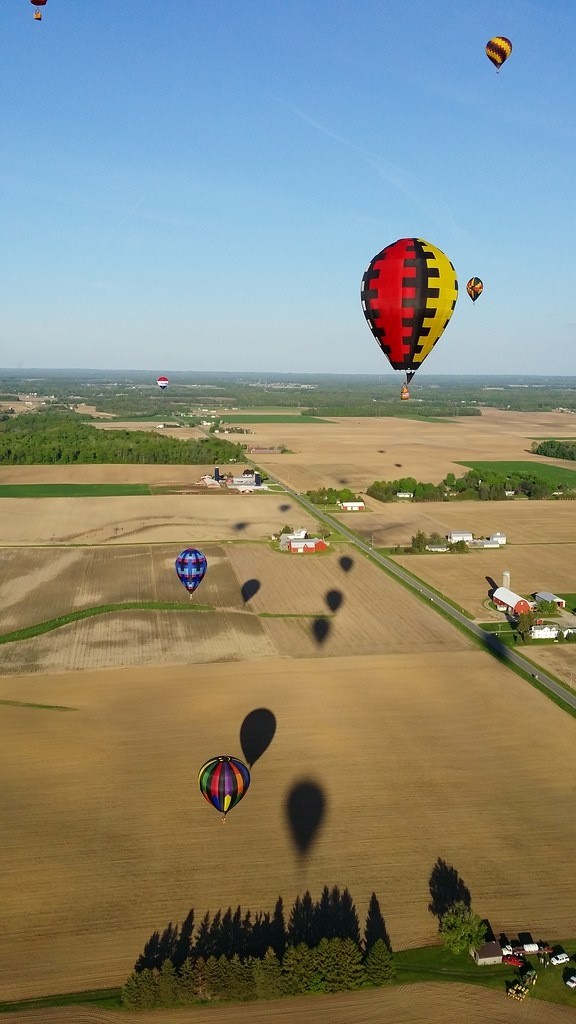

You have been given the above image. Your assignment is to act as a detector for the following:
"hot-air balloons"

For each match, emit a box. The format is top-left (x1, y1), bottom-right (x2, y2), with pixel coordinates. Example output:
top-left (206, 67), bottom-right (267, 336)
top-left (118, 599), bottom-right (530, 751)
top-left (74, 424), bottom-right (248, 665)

top-left (485, 36), bottom-right (512, 74)
top-left (158, 377), bottom-right (169, 394)
top-left (360, 238), bottom-right (459, 400)
top-left (466, 277), bottom-right (484, 305)
top-left (175, 548), bottom-right (208, 602)
top-left (30, 0), bottom-right (48, 21)
top-left (197, 755), bottom-right (251, 823)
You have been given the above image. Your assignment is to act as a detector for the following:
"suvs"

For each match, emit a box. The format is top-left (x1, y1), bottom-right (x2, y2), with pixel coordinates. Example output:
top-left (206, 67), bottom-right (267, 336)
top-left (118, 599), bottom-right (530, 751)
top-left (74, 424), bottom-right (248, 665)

top-left (550, 953), bottom-right (570, 966)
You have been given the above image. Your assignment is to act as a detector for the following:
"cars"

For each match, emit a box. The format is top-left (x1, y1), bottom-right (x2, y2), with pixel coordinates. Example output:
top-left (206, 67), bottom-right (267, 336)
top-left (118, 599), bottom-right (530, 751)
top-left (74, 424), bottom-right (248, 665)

top-left (503, 955), bottom-right (524, 968)
top-left (566, 975), bottom-right (576, 989)
